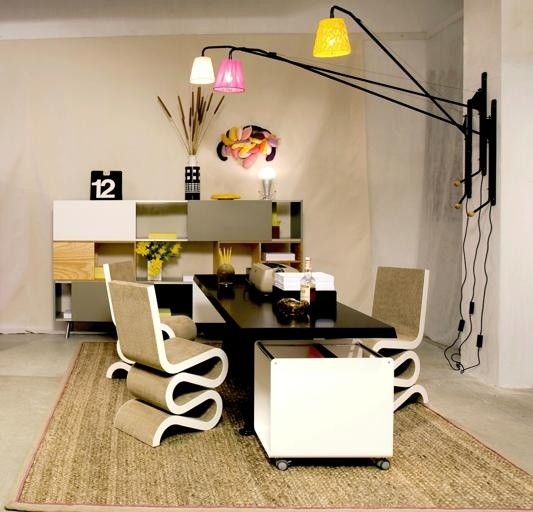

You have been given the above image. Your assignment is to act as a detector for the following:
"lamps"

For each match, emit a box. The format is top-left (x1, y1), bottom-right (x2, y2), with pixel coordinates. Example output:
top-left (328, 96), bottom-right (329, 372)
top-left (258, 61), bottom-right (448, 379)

top-left (190, 44), bottom-right (497, 217)
top-left (312, 4), bottom-right (471, 201)
top-left (212, 46), bottom-right (489, 187)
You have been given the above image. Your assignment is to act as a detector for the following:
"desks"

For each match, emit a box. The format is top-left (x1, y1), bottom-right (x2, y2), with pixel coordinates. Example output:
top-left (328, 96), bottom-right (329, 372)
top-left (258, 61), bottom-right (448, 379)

top-left (191, 275), bottom-right (394, 436)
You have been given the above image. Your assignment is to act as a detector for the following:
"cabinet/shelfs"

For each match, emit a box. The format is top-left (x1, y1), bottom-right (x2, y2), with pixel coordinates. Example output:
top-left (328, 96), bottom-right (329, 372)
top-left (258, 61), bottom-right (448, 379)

top-left (253, 340), bottom-right (395, 472)
top-left (50, 199), bottom-right (302, 340)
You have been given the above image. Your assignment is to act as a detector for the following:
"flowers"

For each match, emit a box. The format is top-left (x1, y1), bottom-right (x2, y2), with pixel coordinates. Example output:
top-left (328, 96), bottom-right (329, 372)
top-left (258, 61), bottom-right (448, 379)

top-left (134, 240), bottom-right (183, 277)
top-left (217, 122), bottom-right (279, 169)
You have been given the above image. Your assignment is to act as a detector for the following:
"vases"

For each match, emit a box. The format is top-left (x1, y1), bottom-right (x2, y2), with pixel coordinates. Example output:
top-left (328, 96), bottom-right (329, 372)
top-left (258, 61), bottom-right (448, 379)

top-left (147, 260), bottom-right (163, 283)
top-left (239, 153), bottom-right (257, 199)
top-left (183, 154), bottom-right (201, 199)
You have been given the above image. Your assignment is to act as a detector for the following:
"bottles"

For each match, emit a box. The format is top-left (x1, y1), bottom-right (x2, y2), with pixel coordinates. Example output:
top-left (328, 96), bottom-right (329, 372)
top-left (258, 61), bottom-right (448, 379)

top-left (299, 256), bottom-right (316, 309)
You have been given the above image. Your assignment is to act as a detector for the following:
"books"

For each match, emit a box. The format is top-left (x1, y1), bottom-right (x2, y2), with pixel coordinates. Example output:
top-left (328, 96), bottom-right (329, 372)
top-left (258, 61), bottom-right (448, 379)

top-left (62, 309), bottom-right (70, 318)
top-left (261, 251), bottom-right (296, 262)
top-left (148, 231), bottom-right (177, 239)
top-left (158, 307), bottom-right (171, 316)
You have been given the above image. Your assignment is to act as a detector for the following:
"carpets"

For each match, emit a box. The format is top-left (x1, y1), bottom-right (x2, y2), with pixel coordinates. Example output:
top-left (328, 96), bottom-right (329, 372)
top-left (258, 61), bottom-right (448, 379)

top-left (4, 341), bottom-right (533, 511)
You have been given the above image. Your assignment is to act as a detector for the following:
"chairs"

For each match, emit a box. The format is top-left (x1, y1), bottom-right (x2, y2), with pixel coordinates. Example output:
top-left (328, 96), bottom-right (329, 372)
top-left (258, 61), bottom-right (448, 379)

top-left (102, 260), bottom-right (198, 378)
top-left (333, 265), bottom-right (430, 413)
top-left (105, 279), bottom-right (229, 448)
